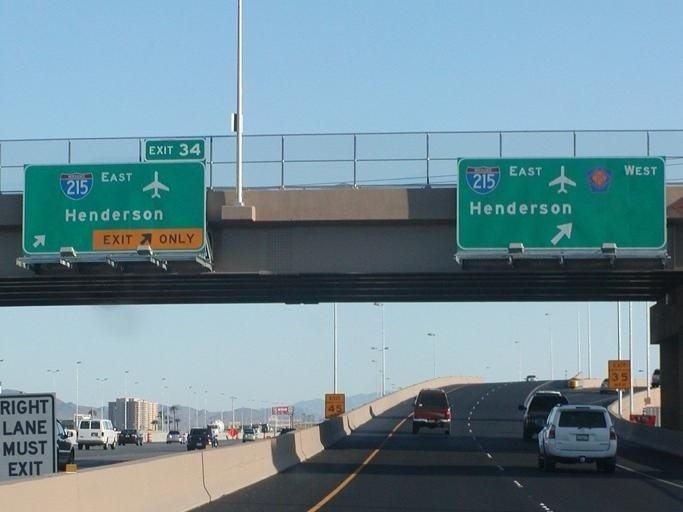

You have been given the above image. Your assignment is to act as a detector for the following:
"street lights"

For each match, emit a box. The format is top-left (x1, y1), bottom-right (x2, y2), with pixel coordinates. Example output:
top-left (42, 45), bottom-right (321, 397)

top-left (160, 378), bottom-right (293, 437)
top-left (125, 370), bottom-right (130, 430)
top-left (96, 377), bottom-right (109, 419)
top-left (46, 369), bottom-right (61, 393)
top-left (76, 359), bottom-right (82, 423)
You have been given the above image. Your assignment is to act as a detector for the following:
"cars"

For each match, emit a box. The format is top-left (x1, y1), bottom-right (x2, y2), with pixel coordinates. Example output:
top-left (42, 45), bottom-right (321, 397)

top-left (242, 428), bottom-right (256, 443)
top-left (651, 369), bottom-right (661, 388)
top-left (55, 417), bottom-right (76, 470)
top-left (599, 378), bottom-right (625, 395)
top-left (166, 430), bottom-right (186, 445)
top-left (118, 428), bottom-right (144, 446)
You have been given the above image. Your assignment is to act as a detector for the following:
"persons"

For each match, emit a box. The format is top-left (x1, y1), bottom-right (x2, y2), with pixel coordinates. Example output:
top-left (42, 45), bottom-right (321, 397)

top-left (136, 425), bottom-right (144, 448)
top-left (210, 430), bottom-right (217, 448)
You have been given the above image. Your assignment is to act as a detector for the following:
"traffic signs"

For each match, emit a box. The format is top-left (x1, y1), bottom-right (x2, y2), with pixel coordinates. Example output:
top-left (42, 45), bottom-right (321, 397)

top-left (454, 154), bottom-right (668, 258)
top-left (21, 160), bottom-right (208, 261)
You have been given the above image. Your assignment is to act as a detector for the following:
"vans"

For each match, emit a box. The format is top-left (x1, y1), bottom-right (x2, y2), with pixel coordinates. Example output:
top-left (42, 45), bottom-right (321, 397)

top-left (526, 375), bottom-right (537, 382)
top-left (186, 427), bottom-right (219, 451)
top-left (411, 388), bottom-right (451, 435)
top-left (77, 418), bottom-right (118, 451)
top-left (517, 389), bottom-right (568, 440)
top-left (536, 403), bottom-right (617, 473)
